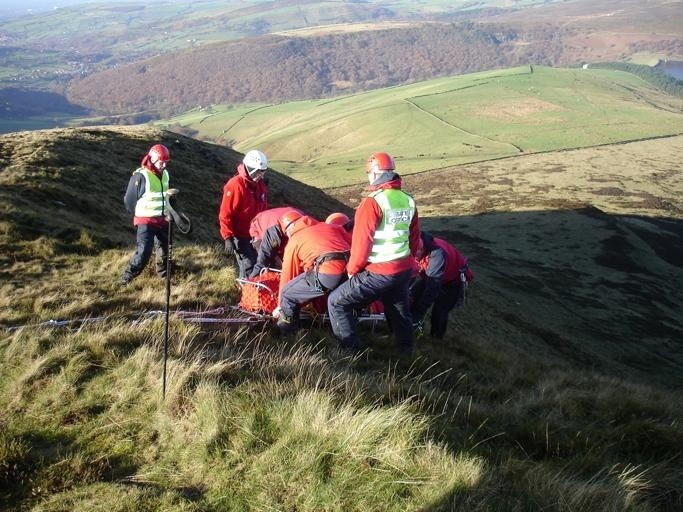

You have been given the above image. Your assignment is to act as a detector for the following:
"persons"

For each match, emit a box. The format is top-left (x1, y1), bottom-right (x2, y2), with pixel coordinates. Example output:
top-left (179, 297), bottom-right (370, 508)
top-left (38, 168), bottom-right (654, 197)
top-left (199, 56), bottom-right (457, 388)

top-left (120, 144), bottom-right (184, 283)
top-left (328, 152), bottom-right (419, 355)
top-left (218, 150), bottom-right (268, 278)
top-left (416, 231), bottom-right (473, 340)
top-left (247, 207), bottom-right (424, 343)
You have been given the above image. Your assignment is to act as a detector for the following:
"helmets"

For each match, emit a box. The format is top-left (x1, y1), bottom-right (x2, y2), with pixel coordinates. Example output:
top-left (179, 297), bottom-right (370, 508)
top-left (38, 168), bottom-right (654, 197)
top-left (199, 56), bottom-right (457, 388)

top-left (280, 212), bottom-right (303, 235)
top-left (324, 213), bottom-right (352, 227)
top-left (147, 144), bottom-right (170, 164)
top-left (366, 152), bottom-right (395, 173)
top-left (242, 149), bottom-right (268, 171)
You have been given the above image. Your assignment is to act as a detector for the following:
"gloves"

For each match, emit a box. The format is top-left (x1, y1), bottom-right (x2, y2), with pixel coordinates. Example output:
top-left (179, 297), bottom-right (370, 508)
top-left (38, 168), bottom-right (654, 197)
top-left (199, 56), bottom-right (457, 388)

top-left (224, 239), bottom-right (237, 256)
